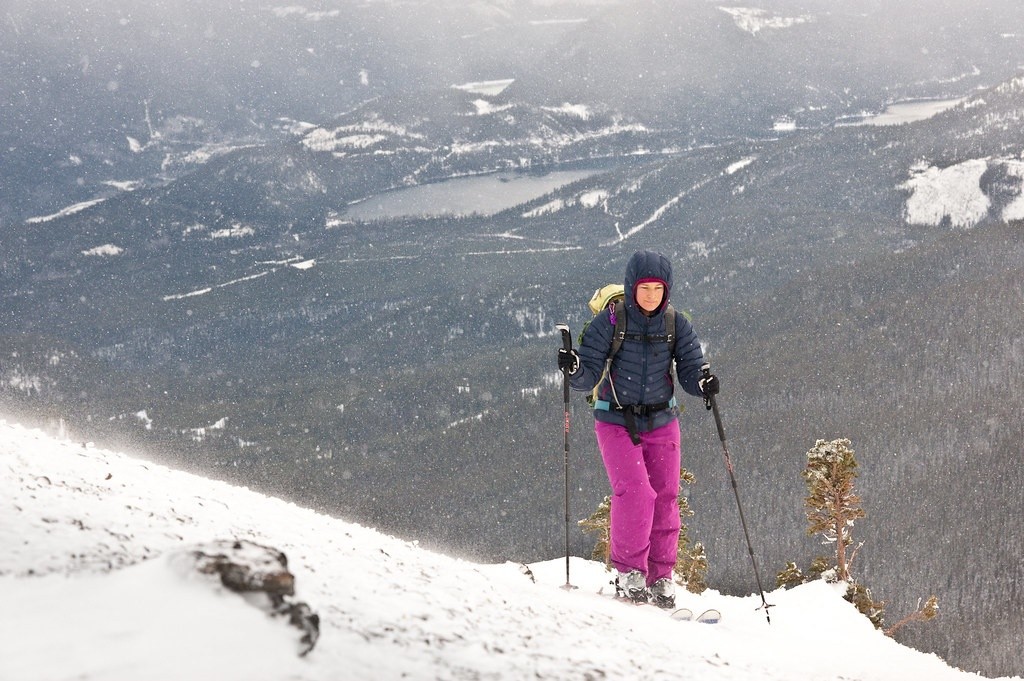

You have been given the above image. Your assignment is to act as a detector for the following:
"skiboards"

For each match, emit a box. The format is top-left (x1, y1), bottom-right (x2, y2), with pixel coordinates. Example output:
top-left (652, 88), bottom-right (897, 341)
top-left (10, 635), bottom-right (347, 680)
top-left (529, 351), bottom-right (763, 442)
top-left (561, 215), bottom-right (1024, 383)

top-left (600, 569), bottom-right (724, 624)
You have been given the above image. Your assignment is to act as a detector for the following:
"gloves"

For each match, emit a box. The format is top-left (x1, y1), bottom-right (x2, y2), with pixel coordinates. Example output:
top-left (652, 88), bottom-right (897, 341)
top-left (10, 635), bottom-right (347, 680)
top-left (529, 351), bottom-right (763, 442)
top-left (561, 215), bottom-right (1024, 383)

top-left (557, 348), bottom-right (582, 377)
top-left (698, 372), bottom-right (719, 396)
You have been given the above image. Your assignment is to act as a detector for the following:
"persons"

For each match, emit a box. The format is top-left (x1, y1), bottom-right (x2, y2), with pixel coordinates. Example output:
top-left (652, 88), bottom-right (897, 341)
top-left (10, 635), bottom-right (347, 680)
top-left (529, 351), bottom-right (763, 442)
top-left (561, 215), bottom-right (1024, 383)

top-left (557, 249), bottom-right (719, 606)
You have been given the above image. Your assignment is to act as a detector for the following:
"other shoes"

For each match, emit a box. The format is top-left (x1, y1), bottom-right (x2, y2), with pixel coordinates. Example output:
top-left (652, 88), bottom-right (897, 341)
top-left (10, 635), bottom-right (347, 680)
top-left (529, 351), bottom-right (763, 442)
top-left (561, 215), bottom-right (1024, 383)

top-left (616, 575), bottom-right (648, 603)
top-left (648, 580), bottom-right (675, 609)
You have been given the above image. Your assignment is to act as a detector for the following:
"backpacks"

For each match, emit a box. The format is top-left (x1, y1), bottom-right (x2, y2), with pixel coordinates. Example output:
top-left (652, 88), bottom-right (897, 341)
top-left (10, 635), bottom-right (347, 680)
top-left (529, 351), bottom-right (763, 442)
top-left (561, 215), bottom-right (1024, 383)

top-left (588, 285), bottom-right (676, 381)
